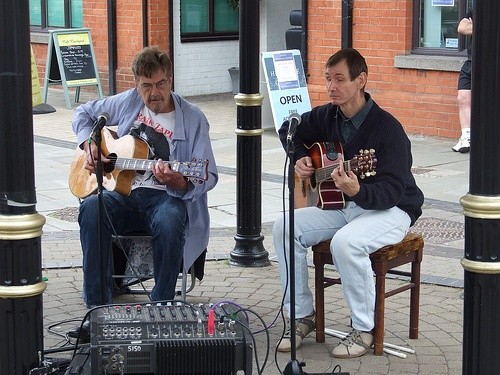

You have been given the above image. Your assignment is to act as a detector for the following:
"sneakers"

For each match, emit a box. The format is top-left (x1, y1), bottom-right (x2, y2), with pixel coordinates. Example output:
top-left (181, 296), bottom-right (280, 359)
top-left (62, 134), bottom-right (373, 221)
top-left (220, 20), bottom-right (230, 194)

top-left (275, 310), bottom-right (315, 351)
top-left (329, 329), bottom-right (374, 357)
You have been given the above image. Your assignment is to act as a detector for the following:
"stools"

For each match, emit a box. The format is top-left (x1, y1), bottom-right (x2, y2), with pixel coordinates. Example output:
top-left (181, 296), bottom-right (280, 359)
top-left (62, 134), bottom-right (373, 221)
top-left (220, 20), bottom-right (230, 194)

top-left (311, 232), bottom-right (424, 357)
top-left (111, 230), bottom-right (195, 306)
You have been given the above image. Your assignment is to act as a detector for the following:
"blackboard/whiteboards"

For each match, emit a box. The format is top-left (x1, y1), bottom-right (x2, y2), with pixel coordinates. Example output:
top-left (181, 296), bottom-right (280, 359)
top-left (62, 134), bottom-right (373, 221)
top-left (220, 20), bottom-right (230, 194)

top-left (45, 27), bottom-right (101, 87)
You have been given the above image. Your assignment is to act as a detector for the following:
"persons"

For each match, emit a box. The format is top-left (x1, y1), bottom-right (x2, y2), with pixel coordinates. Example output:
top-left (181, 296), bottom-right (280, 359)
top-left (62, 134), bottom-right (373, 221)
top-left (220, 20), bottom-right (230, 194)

top-left (275, 48), bottom-right (425, 358)
top-left (452, 11), bottom-right (472, 153)
top-left (67, 45), bottom-right (219, 343)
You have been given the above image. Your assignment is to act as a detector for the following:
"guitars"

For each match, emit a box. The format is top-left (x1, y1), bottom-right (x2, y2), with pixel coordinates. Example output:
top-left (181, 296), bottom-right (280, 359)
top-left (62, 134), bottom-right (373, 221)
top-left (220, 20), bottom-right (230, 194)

top-left (293, 139), bottom-right (378, 210)
top-left (69, 127), bottom-right (209, 199)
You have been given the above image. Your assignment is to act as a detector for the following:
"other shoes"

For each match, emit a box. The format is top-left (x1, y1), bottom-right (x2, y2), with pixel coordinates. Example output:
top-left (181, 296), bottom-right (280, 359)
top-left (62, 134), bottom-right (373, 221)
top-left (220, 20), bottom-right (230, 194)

top-left (452, 143), bottom-right (462, 152)
top-left (67, 319), bottom-right (91, 338)
top-left (458, 136), bottom-right (470, 152)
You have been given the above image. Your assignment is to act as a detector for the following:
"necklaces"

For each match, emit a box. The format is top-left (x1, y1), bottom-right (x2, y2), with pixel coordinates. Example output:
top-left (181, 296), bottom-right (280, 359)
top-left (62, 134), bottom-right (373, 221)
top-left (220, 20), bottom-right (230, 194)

top-left (150, 111), bottom-right (158, 125)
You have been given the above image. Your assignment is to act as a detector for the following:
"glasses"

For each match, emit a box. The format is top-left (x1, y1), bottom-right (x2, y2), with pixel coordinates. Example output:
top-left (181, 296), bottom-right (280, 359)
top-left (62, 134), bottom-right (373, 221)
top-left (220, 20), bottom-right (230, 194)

top-left (137, 78), bottom-right (169, 93)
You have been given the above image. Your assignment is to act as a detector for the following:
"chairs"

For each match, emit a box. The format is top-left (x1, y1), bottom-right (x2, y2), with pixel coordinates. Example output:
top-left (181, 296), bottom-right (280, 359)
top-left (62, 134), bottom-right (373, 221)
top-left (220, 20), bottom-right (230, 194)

top-left (286, 10), bottom-right (302, 50)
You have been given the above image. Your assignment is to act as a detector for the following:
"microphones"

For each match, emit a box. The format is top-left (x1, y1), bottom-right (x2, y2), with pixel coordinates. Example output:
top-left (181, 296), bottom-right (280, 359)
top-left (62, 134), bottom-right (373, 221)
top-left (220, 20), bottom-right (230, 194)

top-left (287, 113), bottom-right (302, 145)
top-left (90, 112), bottom-right (110, 139)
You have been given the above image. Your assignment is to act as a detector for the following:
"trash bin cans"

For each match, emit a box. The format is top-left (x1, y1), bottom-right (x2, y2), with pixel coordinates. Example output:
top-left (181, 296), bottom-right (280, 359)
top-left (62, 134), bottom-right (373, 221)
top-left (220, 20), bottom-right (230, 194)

top-left (228, 66), bottom-right (240, 95)
